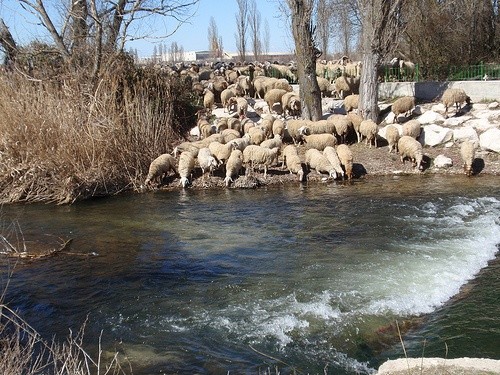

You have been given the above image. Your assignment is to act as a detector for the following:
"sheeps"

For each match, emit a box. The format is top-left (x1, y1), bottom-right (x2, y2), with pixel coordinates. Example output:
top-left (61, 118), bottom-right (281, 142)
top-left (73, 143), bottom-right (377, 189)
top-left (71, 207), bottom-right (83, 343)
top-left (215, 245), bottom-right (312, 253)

top-left (142, 54), bottom-right (424, 188)
top-left (459, 140), bottom-right (476, 178)
top-left (437, 88), bottom-right (471, 116)
